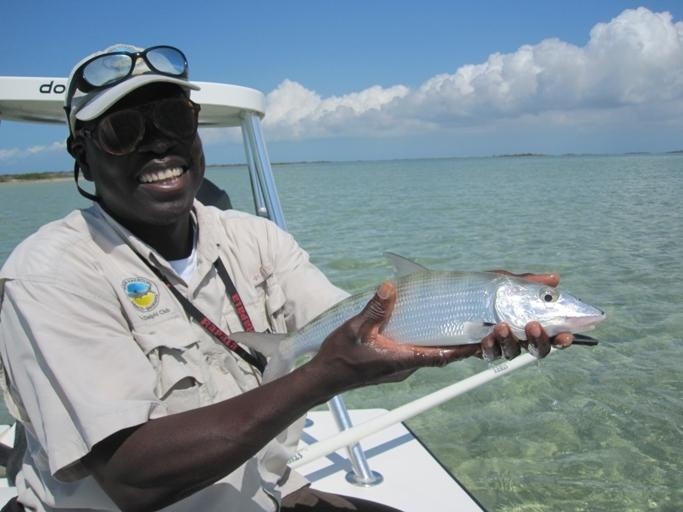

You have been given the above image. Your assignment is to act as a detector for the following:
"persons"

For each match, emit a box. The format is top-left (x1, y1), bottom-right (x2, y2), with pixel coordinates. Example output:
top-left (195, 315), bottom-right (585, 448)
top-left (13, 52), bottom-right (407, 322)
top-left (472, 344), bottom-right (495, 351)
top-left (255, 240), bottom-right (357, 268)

top-left (0, 44), bottom-right (572, 511)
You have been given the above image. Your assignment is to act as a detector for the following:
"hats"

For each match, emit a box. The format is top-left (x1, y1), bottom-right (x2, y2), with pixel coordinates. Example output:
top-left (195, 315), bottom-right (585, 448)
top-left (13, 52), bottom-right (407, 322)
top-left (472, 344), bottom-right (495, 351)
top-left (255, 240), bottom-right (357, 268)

top-left (64, 43), bottom-right (200, 138)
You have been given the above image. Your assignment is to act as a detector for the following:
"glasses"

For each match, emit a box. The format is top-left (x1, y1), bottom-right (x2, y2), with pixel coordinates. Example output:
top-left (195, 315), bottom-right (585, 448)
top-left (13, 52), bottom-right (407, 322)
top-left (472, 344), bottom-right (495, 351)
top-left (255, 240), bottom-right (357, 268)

top-left (66, 44), bottom-right (187, 111)
top-left (74, 99), bottom-right (200, 155)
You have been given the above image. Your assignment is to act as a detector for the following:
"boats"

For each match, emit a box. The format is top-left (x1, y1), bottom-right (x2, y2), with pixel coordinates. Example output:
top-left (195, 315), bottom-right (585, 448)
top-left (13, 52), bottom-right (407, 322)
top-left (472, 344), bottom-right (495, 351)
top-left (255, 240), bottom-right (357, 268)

top-left (0, 74), bottom-right (486, 512)
